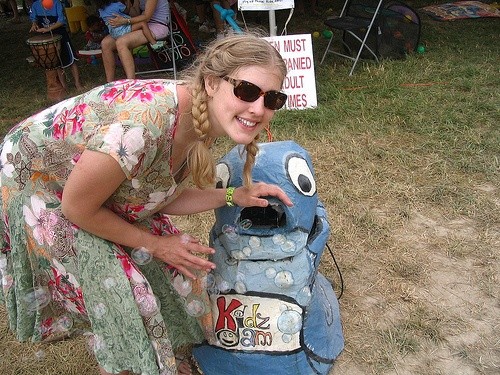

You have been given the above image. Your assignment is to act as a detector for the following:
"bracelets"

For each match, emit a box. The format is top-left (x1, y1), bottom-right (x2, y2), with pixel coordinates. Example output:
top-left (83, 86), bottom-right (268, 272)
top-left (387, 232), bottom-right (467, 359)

top-left (127, 19), bottom-right (130, 25)
top-left (225, 186), bottom-right (236, 208)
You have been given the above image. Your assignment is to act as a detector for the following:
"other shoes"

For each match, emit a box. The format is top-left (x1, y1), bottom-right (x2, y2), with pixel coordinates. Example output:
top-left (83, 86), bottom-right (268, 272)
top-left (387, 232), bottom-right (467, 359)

top-left (150, 40), bottom-right (167, 50)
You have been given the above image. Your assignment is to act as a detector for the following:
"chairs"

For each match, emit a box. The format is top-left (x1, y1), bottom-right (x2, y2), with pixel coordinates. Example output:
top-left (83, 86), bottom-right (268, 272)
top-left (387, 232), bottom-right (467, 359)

top-left (135, 9), bottom-right (181, 80)
top-left (319, 0), bottom-right (383, 77)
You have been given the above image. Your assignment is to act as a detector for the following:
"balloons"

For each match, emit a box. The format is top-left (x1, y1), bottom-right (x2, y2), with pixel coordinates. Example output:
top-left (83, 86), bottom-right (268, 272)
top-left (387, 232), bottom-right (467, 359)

top-left (214, 4), bottom-right (242, 35)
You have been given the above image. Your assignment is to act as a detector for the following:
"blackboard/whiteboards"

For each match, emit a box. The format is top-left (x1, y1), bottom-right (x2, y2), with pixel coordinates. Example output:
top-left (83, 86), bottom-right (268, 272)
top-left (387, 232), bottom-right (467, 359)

top-left (150, 6), bottom-right (195, 73)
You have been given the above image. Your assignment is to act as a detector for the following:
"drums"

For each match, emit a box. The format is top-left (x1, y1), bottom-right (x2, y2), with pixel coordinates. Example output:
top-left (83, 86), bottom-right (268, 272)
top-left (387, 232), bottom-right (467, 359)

top-left (26, 34), bottom-right (70, 101)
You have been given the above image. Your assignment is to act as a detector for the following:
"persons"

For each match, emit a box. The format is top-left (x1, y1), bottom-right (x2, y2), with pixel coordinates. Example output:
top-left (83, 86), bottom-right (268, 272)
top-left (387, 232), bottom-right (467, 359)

top-left (84, 15), bottom-right (101, 53)
top-left (196, 0), bottom-right (225, 40)
top-left (100, 0), bottom-right (172, 85)
top-left (96, 0), bottom-right (168, 53)
top-left (30, 0), bottom-right (86, 93)
top-left (0, 32), bottom-right (292, 375)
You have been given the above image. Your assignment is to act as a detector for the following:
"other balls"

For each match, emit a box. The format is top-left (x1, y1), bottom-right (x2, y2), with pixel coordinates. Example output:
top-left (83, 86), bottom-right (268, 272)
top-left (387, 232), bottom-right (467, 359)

top-left (325, 31), bottom-right (333, 39)
top-left (313, 32), bottom-right (320, 38)
top-left (323, 29), bottom-right (328, 35)
top-left (417, 45), bottom-right (425, 54)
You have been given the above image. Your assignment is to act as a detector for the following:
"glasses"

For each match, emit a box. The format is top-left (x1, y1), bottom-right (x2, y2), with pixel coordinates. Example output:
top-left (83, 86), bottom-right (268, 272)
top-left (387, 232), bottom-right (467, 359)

top-left (218, 74), bottom-right (288, 110)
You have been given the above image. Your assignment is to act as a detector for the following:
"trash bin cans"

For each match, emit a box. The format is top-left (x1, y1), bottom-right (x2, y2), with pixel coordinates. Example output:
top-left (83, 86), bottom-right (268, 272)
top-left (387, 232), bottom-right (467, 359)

top-left (190, 140), bottom-right (345, 375)
